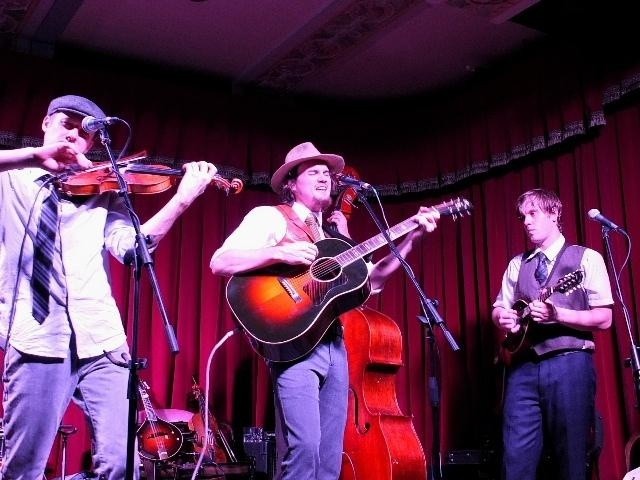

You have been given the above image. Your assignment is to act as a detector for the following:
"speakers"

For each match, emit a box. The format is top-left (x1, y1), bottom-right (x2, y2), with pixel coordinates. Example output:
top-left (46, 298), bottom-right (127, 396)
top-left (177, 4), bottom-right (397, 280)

top-left (243, 433), bottom-right (276, 480)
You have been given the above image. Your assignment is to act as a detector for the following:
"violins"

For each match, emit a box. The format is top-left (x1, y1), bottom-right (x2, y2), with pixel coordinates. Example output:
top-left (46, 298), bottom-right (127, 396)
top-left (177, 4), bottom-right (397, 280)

top-left (58, 163), bottom-right (242, 197)
top-left (188, 376), bottom-right (230, 468)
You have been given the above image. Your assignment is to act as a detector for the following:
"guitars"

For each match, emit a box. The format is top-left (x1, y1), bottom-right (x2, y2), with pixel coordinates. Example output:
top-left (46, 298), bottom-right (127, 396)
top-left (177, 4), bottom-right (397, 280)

top-left (499, 272), bottom-right (584, 354)
top-left (226, 196), bottom-right (475, 361)
top-left (137, 379), bottom-right (183, 461)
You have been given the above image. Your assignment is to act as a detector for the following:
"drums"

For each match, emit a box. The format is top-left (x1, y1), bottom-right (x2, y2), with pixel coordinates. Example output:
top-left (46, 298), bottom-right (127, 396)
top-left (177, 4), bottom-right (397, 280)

top-left (135, 409), bottom-right (198, 479)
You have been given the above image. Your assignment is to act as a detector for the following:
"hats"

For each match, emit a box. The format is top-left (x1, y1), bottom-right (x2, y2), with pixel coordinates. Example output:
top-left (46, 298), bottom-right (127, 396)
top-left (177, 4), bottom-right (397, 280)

top-left (47, 95), bottom-right (107, 120)
top-left (271, 142), bottom-right (345, 195)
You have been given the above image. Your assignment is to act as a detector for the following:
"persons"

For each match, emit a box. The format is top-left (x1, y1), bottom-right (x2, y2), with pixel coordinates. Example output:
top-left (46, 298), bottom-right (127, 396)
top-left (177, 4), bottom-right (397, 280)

top-left (325, 208), bottom-right (354, 243)
top-left (488, 188), bottom-right (617, 480)
top-left (210, 140), bottom-right (441, 480)
top-left (0, 94), bottom-right (220, 480)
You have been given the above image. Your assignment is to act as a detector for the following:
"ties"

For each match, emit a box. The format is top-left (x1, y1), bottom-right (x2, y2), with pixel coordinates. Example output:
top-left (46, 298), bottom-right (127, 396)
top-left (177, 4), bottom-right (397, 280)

top-left (534, 252), bottom-right (548, 285)
top-left (305, 213), bottom-right (321, 241)
top-left (32, 174), bottom-right (68, 325)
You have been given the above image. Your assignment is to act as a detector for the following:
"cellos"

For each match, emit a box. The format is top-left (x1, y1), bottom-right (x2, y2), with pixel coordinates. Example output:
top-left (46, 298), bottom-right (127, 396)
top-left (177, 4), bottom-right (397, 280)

top-left (323, 167), bottom-right (426, 480)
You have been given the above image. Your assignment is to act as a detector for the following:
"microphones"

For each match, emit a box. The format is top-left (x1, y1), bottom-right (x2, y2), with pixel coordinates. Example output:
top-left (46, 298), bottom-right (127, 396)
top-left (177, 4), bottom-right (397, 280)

top-left (81, 116), bottom-right (119, 134)
top-left (331, 172), bottom-right (371, 189)
top-left (587, 209), bottom-right (627, 235)
